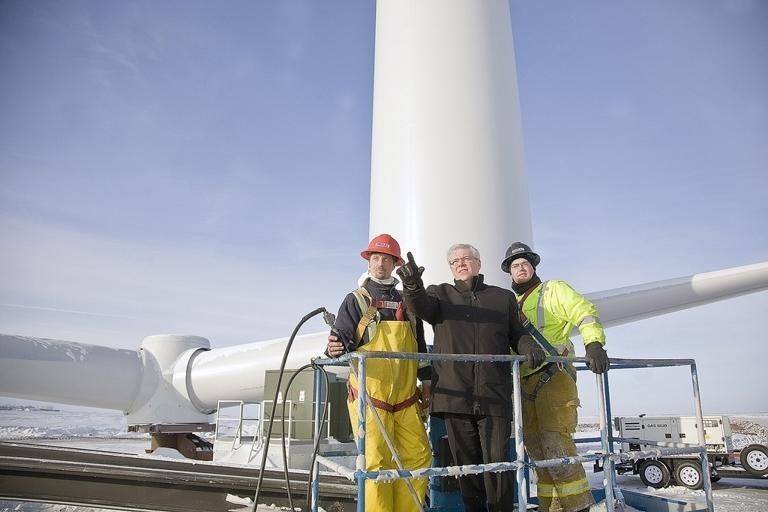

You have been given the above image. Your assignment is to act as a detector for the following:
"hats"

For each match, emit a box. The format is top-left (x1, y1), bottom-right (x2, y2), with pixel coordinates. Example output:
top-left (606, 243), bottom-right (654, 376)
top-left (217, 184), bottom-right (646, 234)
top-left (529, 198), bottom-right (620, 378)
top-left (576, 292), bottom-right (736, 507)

top-left (360, 234), bottom-right (405, 267)
top-left (500, 242), bottom-right (541, 274)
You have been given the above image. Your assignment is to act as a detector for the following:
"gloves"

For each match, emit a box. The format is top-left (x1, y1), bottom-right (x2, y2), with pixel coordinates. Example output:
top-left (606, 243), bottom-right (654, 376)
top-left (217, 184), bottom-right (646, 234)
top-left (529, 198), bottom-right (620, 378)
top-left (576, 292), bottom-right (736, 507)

top-left (396, 252), bottom-right (425, 291)
top-left (584, 341), bottom-right (610, 375)
top-left (517, 334), bottom-right (547, 370)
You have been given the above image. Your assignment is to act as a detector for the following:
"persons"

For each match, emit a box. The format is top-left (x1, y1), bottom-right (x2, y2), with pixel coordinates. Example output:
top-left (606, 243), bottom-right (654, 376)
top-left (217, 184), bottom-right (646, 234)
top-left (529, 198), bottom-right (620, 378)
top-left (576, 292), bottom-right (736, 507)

top-left (324, 233), bottom-right (435, 511)
top-left (500, 241), bottom-right (609, 511)
top-left (395, 242), bottom-right (547, 511)
